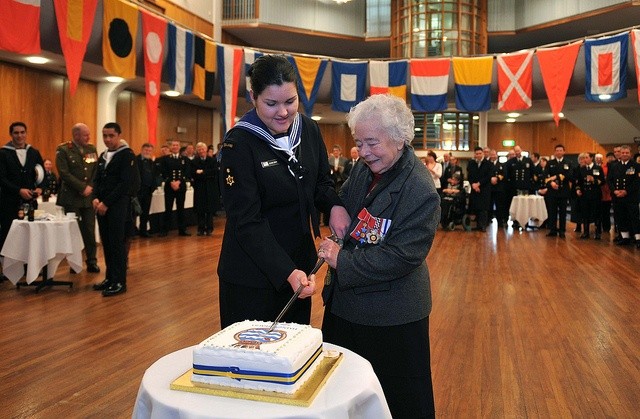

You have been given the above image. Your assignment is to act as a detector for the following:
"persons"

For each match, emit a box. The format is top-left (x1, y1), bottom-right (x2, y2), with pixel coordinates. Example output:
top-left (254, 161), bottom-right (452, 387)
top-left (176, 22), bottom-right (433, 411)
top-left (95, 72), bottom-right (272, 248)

top-left (0, 122), bottom-right (48, 257)
top-left (342, 146), bottom-right (359, 179)
top-left (136, 138), bottom-right (219, 238)
top-left (328, 144), bottom-right (349, 194)
top-left (55, 122), bottom-right (101, 273)
top-left (216, 54), bottom-right (352, 332)
top-left (317, 94), bottom-right (441, 418)
top-left (442, 145), bottom-right (545, 232)
top-left (90, 122), bottom-right (136, 297)
top-left (425, 150), bottom-right (443, 197)
top-left (545, 143), bottom-right (639, 246)
top-left (42, 159), bottom-right (57, 196)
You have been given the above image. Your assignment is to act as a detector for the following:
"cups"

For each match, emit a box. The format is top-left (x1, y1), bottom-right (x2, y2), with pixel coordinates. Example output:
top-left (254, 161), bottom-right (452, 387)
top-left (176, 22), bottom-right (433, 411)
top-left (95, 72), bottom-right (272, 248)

top-left (56, 206), bottom-right (63, 220)
top-left (67, 212), bottom-right (75, 221)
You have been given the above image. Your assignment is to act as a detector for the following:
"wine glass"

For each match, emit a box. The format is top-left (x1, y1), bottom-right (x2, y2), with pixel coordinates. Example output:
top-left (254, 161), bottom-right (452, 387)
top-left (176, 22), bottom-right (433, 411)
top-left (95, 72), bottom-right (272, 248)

top-left (23, 204), bottom-right (28, 219)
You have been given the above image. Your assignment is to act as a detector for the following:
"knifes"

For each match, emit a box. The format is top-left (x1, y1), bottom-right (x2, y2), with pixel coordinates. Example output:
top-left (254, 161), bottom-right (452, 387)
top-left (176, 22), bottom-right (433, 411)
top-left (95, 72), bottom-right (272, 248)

top-left (268, 233), bottom-right (344, 333)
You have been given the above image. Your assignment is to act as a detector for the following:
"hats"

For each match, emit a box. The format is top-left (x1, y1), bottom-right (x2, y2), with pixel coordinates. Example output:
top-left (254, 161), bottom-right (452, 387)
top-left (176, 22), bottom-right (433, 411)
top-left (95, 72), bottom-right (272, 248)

top-left (34, 163), bottom-right (45, 185)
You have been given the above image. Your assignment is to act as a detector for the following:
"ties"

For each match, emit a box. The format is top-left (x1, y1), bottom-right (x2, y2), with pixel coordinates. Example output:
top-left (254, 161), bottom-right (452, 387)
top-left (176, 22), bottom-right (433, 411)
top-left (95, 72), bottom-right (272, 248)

top-left (623, 162), bottom-right (625, 168)
top-left (559, 160), bottom-right (560, 165)
top-left (518, 158), bottom-right (521, 163)
top-left (174, 154), bottom-right (176, 161)
top-left (587, 166), bottom-right (590, 170)
top-left (478, 161), bottom-right (480, 168)
top-left (202, 158), bottom-right (205, 163)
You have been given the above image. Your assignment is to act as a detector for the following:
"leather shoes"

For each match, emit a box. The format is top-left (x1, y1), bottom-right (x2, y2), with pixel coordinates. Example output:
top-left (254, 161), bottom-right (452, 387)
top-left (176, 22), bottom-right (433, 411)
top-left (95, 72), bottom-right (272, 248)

top-left (617, 240), bottom-right (628, 245)
top-left (482, 225), bottom-right (486, 232)
top-left (546, 232), bottom-right (557, 236)
top-left (206, 231), bottom-right (212, 235)
top-left (559, 233), bottom-right (565, 238)
top-left (93, 279), bottom-right (109, 290)
top-left (580, 233), bottom-right (589, 238)
top-left (140, 232), bottom-right (152, 237)
top-left (102, 282), bottom-right (126, 296)
top-left (87, 265), bottom-right (100, 272)
top-left (635, 241), bottom-right (640, 248)
top-left (198, 231), bottom-right (204, 236)
top-left (179, 228), bottom-right (192, 236)
top-left (158, 230), bottom-right (169, 236)
top-left (594, 234), bottom-right (600, 239)
top-left (573, 228), bottom-right (581, 232)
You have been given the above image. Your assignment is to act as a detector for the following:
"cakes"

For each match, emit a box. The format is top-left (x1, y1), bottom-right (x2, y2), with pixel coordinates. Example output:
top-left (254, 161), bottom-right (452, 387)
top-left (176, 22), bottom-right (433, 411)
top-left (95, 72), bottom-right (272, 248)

top-left (190, 317), bottom-right (326, 395)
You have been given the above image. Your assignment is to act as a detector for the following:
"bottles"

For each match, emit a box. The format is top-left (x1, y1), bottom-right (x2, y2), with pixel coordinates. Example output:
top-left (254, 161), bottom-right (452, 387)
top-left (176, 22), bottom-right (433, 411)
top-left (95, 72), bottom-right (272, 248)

top-left (17, 199), bottom-right (24, 220)
top-left (27, 198), bottom-right (35, 221)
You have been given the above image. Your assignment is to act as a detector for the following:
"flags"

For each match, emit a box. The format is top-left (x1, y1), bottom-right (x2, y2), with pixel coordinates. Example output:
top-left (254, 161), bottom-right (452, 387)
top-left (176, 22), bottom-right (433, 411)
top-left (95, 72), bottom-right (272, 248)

top-left (168, 21), bottom-right (194, 93)
top-left (330, 57), bottom-right (368, 112)
top-left (244, 48), bottom-right (277, 103)
top-left (369, 60), bottom-right (408, 104)
top-left (497, 50), bottom-right (534, 112)
top-left (0, 0), bottom-right (42, 55)
top-left (191, 32), bottom-right (217, 103)
top-left (452, 57), bottom-right (494, 113)
top-left (102, 0), bottom-right (140, 81)
top-left (631, 29), bottom-right (640, 103)
top-left (583, 31), bottom-right (629, 103)
top-left (410, 58), bottom-right (451, 112)
